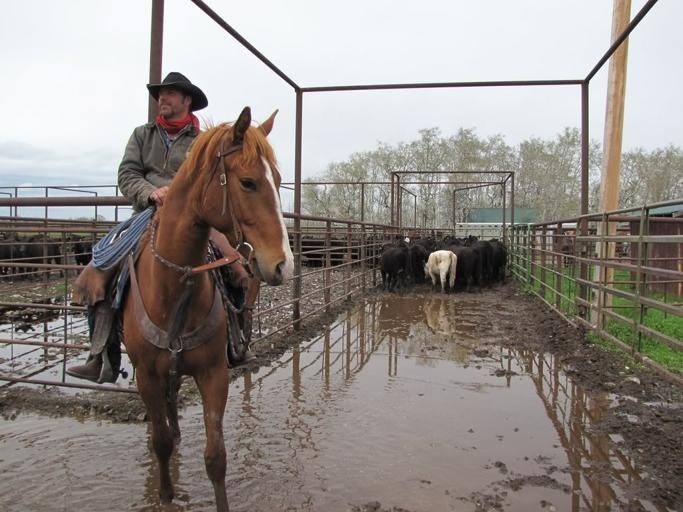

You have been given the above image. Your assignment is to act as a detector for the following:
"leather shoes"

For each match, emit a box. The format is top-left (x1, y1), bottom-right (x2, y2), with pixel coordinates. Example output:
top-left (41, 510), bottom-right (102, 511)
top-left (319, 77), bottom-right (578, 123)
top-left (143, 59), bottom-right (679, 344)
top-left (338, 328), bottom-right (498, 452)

top-left (229, 344), bottom-right (258, 369)
top-left (65, 356), bottom-right (120, 383)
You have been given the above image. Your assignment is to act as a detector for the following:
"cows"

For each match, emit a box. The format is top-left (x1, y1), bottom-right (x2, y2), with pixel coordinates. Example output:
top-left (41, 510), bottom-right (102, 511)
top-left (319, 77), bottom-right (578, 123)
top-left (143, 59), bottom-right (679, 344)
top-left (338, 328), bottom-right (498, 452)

top-left (288, 234), bottom-right (508, 297)
top-left (0, 228), bottom-right (99, 282)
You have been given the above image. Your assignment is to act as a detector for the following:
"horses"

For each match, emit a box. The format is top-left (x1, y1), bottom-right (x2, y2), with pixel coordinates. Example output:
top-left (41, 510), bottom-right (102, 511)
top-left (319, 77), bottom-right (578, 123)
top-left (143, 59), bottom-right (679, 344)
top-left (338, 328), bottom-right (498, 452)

top-left (119, 105), bottom-right (295, 512)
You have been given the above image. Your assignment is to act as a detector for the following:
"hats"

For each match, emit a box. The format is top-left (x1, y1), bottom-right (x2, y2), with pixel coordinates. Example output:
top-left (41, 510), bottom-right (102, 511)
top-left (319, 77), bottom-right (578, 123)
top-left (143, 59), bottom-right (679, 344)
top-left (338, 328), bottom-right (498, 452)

top-left (147, 72), bottom-right (208, 113)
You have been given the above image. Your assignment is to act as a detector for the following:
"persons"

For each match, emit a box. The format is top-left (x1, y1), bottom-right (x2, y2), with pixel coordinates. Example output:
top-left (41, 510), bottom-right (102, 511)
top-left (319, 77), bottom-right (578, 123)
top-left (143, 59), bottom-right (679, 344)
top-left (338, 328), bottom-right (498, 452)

top-left (63, 71), bottom-right (259, 385)
